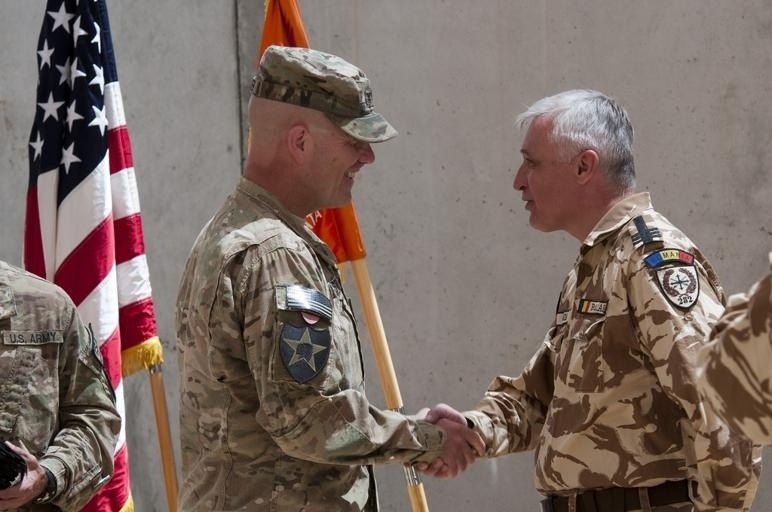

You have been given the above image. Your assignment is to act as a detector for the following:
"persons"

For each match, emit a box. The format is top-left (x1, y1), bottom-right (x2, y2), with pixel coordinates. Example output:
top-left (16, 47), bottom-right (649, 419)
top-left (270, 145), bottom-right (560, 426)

top-left (176, 43), bottom-right (487, 511)
top-left (0, 259), bottom-right (122, 511)
top-left (691, 251), bottom-right (772, 446)
top-left (417, 87), bottom-right (764, 511)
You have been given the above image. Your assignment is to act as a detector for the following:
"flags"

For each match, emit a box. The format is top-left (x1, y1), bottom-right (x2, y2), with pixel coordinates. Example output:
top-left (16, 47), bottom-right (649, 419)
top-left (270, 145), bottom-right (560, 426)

top-left (20, 0), bottom-right (165, 511)
top-left (248, 1), bottom-right (363, 285)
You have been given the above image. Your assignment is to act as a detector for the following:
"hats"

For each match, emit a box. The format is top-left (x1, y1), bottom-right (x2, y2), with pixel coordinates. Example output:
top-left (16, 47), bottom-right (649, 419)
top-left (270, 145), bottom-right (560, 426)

top-left (251, 44), bottom-right (397, 143)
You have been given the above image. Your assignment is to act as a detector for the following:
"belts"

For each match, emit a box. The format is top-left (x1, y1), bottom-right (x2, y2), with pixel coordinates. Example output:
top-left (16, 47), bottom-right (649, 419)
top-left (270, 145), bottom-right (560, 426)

top-left (540, 480), bottom-right (698, 512)
